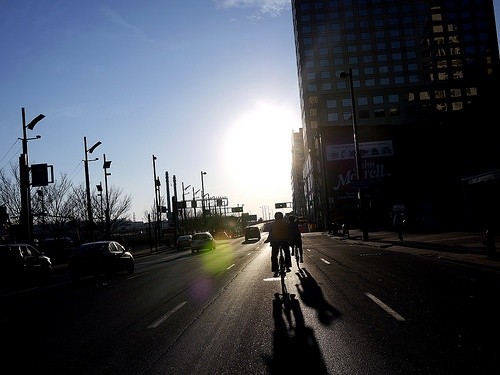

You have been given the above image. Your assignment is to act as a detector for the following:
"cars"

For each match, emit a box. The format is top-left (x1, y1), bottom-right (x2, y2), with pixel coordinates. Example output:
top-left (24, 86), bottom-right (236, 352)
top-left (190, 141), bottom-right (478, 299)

top-left (76, 240), bottom-right (135, 277)
top-left (245, 227), bottom-right (261, 241)
top-left (176, 234), bottom-right (193, 250)
top-left (0, 243), bottom-right (53, 283)
top-left (190, 231), bottom-right (216, 254)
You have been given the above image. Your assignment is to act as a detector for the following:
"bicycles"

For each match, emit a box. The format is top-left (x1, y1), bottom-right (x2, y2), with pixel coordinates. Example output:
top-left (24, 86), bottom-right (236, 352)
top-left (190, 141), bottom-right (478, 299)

top-left (292, 236), bottom-right (303, 268)
top-left (270, 243), bottom-right (292, 295)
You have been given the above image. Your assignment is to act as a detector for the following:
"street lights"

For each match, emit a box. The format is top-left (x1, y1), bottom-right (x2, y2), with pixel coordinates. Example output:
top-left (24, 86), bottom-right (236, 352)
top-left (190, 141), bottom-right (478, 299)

top-left (201, 171), bottom-right (206, 221)
top-left (313, 133), bottom-right (332, 234)
top-left (83, 136), bottom-right (101, 240)
top-left (339, 68), bottom-right (370, 242)
top-left (21, 107), bottom-right (46, 242)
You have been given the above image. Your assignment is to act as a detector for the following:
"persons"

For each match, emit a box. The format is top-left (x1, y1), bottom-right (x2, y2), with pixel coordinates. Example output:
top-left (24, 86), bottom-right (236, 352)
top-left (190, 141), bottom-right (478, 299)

top-left (287, 215), bottom-right (303, 263)
top-left (264, 212), bottom-right (293, 277)
top-left (304, 223), bottom-right (309, 233)
top-left (327, 215), bottom-right (337, 234)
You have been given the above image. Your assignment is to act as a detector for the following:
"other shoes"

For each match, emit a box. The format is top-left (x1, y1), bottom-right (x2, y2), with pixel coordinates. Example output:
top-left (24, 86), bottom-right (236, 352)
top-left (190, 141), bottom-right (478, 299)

top-left (286, 268), bottom-right (291, 271)
top-left (274, 272), bottom-right (278, 276)
